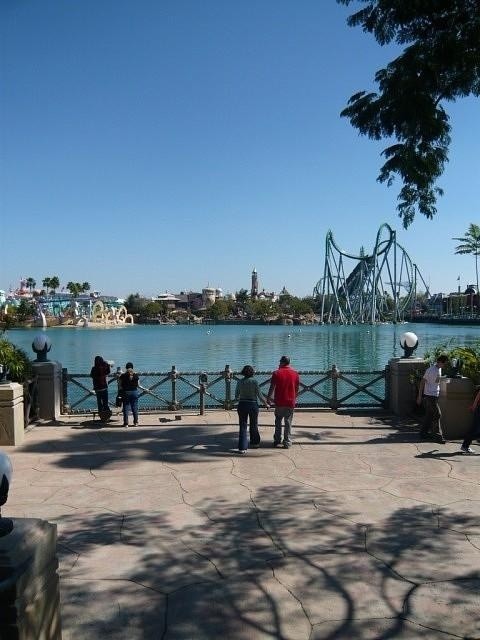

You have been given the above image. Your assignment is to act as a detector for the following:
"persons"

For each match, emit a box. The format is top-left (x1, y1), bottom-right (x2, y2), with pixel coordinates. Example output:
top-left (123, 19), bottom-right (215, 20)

top-left (461, 390), bottom-right (480, 456)
top-left (416, 356), bottom-right (448, 444)
top-left (235, 365), bottom-right (268, 453)
top-left (267, 355), bottom-right (299, 448)
top-left (90, 356), bottom-right (112, 420)
top-left (119, 362), bottom-right (140, 428)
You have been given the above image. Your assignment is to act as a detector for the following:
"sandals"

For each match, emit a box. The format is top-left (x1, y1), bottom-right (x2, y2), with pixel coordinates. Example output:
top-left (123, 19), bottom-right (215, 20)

top-left (238, 448), bottom-right (247, 454)
top-left (250, 442), bottom-right (260, 449)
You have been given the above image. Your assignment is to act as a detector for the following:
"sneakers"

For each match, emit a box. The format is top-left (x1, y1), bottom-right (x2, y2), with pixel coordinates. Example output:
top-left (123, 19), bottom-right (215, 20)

top-left (461, 446), bottom-right (476, 453)
top-left (122, 422), bottom-right (130, 427)
top-left (133, 422), bottom-right (139, 427)
top-left (272, 441), bottom-right (290, 449)
top-left (419, 435), bottom-right (446, 444)
